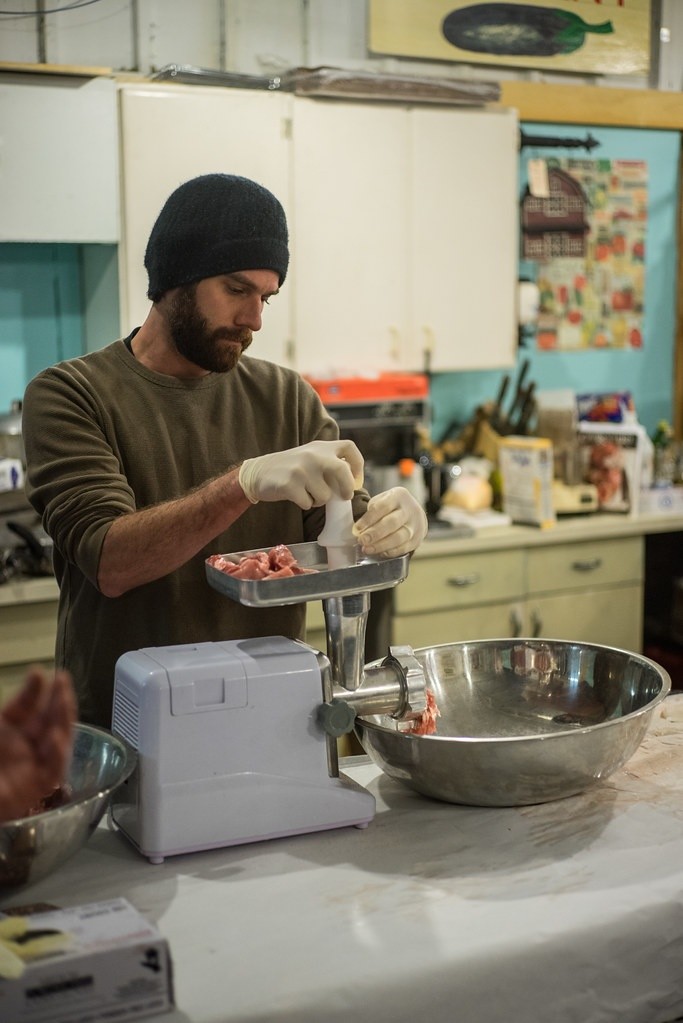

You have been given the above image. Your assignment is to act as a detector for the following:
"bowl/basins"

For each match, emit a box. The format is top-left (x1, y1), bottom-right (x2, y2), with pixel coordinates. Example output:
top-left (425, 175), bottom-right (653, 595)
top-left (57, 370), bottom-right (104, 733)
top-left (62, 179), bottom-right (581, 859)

top-left (0, 722), bottom-right (135, 890)
top-left (351, 637), bottom-right (672, 809)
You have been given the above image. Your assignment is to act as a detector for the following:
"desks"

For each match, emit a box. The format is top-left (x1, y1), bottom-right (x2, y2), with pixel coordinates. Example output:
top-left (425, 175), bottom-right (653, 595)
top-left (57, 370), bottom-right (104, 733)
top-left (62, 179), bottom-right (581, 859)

top-left (0, 690), bottom-right (683, 1023)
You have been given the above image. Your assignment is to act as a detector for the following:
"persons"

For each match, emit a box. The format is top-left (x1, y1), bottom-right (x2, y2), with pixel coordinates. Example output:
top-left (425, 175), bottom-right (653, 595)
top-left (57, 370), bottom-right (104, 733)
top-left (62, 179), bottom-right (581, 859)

top-left (23, 173), bottom-right (429, 734)
top-left (0, 670), bottom-right (78, 827)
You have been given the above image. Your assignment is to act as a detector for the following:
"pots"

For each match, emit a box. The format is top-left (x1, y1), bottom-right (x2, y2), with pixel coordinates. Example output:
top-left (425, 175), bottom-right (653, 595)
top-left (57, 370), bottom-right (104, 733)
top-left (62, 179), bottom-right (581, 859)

top-left (5, 520), bottom-right (54, 576)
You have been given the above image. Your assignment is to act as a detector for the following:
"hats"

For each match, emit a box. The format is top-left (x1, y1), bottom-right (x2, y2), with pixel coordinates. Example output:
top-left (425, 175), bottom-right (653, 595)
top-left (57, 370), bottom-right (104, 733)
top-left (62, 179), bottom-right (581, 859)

top-left (144, 174), bottom-right (291, 301)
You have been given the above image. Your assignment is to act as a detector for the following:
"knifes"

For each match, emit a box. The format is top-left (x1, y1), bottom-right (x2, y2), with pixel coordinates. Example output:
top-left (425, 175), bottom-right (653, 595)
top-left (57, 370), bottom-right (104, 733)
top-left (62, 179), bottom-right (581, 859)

top-left (489, 354), bottom-right (536, 434)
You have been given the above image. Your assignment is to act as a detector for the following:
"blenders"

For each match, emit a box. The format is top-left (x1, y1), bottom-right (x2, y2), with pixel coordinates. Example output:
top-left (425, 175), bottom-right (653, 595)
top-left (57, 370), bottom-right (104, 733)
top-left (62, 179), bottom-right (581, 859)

top-left (112, 537), bottom-right (428, 864)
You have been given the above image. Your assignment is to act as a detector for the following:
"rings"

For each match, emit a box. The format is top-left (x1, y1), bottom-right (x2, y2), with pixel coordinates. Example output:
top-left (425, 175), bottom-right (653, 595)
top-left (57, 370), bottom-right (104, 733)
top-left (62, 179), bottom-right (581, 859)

top-left (404, 525), bottom-right (413, 541)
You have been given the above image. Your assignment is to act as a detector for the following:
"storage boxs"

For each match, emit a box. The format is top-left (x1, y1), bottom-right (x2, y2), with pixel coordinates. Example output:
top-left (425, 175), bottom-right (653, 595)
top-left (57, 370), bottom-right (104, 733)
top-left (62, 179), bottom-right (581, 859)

top-left (576, 421), bottom-right (645, 518)
top-left (500, 433), bottom-right (560, 531)
top-left (0, 896), bottom-right (176, 1023)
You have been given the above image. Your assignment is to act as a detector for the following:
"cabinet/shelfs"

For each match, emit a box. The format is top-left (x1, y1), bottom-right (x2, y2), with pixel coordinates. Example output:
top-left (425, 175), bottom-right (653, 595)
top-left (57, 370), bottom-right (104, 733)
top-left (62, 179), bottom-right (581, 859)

top-left (362, 514), bottom-right (683, 659)
